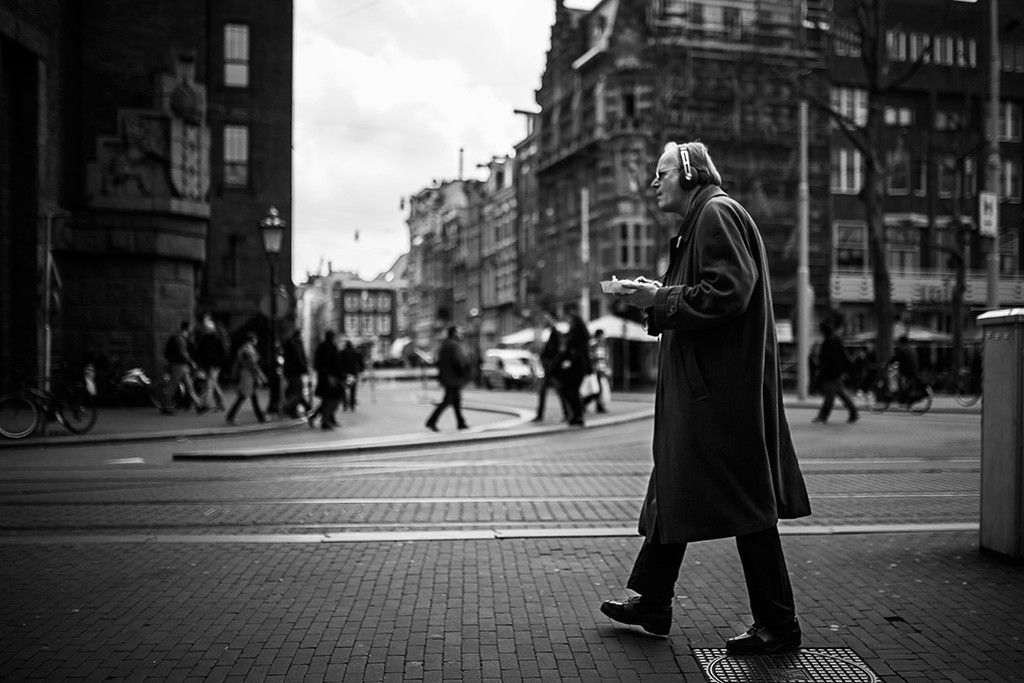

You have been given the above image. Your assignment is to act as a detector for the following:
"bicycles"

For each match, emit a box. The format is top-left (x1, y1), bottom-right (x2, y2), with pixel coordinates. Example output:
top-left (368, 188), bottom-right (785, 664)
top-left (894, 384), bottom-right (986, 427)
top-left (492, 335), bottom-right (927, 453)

top-left (150, 366), bottom-right (223, 413)
top-left (0, 356), bottom-right (98, 440)
top-left (861, 368), bottom-right (980, 416)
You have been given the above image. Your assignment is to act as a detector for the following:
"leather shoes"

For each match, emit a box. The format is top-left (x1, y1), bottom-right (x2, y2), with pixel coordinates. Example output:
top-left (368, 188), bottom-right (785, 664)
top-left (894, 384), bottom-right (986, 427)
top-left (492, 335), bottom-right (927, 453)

top-left (725, 616), bottom-right (802, 653)
top-left (601, 596), bottom-right (672, 635)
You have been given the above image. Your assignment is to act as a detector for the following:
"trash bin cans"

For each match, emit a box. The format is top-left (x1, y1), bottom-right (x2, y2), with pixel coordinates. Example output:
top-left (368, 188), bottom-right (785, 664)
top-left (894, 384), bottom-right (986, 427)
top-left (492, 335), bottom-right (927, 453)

top-left (974, 308), bottom-right (1024, 568)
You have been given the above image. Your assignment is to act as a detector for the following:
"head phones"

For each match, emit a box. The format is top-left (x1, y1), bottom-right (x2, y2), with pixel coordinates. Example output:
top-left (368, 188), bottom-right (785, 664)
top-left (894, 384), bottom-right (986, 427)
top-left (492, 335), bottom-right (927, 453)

top-left (678, 143), bottom-right (698, 190)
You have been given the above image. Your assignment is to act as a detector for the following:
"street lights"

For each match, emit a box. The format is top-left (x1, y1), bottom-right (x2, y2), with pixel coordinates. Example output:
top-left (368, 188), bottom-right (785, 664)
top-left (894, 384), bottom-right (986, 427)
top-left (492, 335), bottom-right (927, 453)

top-left (257, 205), bottom-right (286, 417)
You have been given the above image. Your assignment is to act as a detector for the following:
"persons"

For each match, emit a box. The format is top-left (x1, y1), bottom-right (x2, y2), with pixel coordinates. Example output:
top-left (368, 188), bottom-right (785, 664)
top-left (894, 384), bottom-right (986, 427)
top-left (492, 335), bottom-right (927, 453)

top-left (810, 324), bottom-right (982, 425)
top-left (426, 325), bottom-right (474, 433)
top-left (164, 311), bottom-right (363, 432)
top-left (600, 141), bottom-right (813, 650)
top-left (531, 301), bottom-right (611, 428)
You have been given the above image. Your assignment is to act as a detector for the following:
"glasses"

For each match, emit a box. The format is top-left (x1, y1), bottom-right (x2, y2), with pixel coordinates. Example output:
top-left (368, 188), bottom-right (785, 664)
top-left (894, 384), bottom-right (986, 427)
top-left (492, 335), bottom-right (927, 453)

top-left (656, 166), bottom-right (681, 180)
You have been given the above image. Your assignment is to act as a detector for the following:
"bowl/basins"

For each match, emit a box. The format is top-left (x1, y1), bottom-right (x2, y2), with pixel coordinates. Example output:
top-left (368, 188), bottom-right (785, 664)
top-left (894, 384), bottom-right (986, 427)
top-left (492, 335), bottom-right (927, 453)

top-left (600, 281), bottom-right (638, 295)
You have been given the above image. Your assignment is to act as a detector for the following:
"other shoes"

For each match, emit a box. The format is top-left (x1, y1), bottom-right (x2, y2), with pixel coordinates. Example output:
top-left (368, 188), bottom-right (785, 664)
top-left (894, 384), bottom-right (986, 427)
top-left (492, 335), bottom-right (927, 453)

top-left (457, 423), bottom-right (468, 430)
top-left (166, 397), bottom-right (359, 432)
top-left (814, 417), bottom-right (829, 425)
top-left (531, 412), bottom-right (586, 428)
top-left (847, 415), bottom-right (862, 425)
top-left (426, 423), bottom-right (442, 431)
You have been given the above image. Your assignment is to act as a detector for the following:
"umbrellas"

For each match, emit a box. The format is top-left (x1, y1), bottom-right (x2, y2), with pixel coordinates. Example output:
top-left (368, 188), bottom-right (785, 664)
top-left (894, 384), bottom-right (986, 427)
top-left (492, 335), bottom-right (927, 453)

top-left (773, 319), bottom-right (955, 343)
top-left (504, 315), bottom-right (657, 343)
